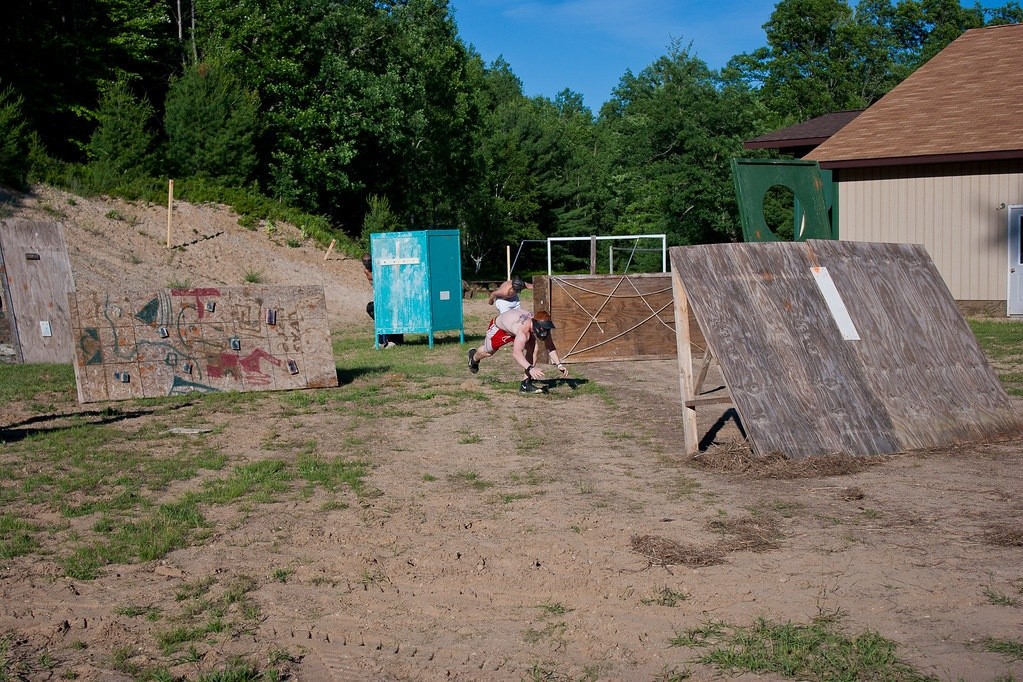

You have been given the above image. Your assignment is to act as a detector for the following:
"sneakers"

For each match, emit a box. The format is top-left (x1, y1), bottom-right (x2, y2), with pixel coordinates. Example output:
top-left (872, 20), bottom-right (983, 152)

top-left (468, 348), bottom-right (481, 374)
top-left (519, 377), bottom-right (546, 393)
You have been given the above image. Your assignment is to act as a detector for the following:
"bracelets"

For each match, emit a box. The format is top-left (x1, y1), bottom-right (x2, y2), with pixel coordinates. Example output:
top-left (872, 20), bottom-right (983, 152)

top-left (557, 363), bottom-right (562, 367)
top-left (527, 366), bottom-right (533, 370)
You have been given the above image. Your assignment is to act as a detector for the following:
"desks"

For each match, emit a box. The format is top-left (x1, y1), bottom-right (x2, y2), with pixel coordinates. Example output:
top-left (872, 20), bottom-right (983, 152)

top-left (470, 281), bottom-right (504, 297)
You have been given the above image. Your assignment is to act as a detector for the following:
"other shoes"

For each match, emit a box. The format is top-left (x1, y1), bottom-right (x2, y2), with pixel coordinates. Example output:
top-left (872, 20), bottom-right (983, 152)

top-left (384, 341), bottom-right (395, 349)
top-left (372, 343), bottom-right (383, 350)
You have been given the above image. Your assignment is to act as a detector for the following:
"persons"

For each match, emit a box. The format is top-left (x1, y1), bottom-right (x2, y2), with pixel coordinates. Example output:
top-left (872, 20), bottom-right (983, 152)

top-left (469, 309), bottom-right (569, 394)
top-left (361, 253), bottom-right (395, 350)
top-left (489, 280), bottom-right (533, 313)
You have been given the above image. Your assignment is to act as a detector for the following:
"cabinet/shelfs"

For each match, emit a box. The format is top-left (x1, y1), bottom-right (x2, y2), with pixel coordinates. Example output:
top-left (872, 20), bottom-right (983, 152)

top-left (370, 229), bottom-right (464, 351)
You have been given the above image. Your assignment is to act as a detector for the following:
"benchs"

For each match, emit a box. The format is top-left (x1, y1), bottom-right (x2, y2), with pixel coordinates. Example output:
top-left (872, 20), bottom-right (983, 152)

top-left (478, 290), bottom-right (496, 292)
